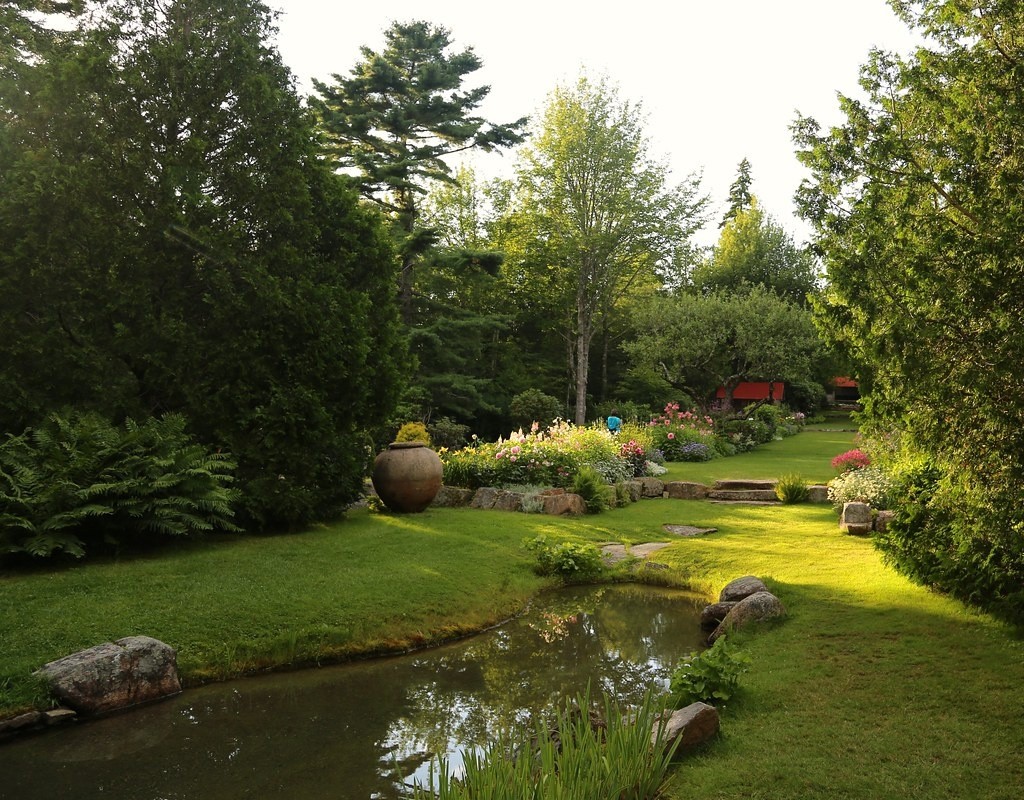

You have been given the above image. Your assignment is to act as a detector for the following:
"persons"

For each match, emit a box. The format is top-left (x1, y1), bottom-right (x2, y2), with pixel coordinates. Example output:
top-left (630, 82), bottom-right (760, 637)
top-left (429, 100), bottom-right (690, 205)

top-left (607, 408), bottom-right (622, 434)
